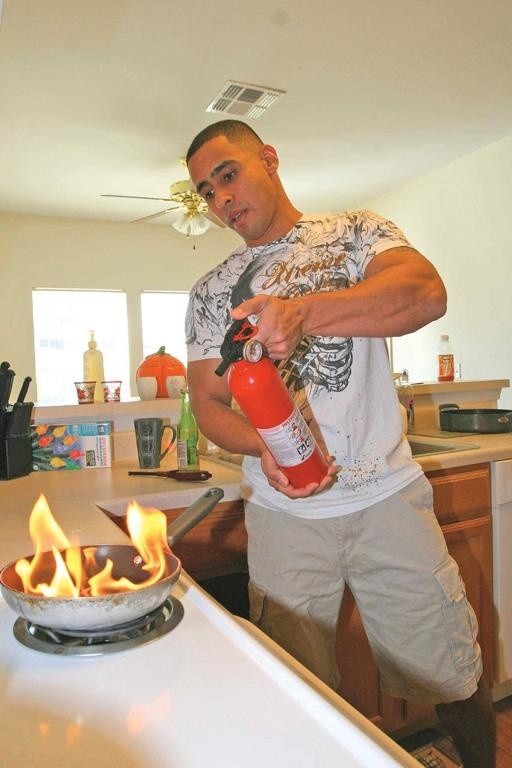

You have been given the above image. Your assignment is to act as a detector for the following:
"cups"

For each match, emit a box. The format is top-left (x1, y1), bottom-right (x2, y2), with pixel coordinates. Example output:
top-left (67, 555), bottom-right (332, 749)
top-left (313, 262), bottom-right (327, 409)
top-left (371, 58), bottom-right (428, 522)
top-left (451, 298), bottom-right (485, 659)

top-left (132, 417), bottom-right (177, 468)
top-left (74, 380), bottom-right (96, 405)
top-left (101, 380), bottom-right (122, 403)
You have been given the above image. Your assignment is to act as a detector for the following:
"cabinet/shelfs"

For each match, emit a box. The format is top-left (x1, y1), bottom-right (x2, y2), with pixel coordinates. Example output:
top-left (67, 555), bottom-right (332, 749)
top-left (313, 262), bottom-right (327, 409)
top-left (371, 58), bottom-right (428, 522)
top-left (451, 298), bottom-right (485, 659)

top-left (108, 460), bottom-right (496, 741)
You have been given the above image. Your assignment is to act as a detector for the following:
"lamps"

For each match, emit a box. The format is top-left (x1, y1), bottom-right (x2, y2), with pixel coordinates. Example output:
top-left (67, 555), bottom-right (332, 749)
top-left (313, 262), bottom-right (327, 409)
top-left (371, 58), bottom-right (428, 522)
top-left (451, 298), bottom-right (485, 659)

top-left (166, 208), bottom-right (211, 251)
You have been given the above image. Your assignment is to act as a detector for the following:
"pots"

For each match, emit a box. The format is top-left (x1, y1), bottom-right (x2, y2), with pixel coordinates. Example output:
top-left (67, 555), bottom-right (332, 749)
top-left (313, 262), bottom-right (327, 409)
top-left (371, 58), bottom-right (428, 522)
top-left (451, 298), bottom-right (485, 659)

top-left (437, 403), bottom-right (512, 433)
top-left (0, 486), bottom-right (226, 631)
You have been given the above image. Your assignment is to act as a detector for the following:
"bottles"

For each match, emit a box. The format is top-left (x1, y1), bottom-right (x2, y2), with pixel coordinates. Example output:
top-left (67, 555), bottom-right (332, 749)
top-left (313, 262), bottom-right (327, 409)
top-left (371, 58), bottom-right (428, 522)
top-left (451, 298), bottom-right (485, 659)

top-left (436, 334), bottom-right (456, 383)
top-left (82, 334), bottom-right (104, 403)
top-left (177, 389), bottom-right (199, 473)
top-left (396, 368), bottom-right (415, 433)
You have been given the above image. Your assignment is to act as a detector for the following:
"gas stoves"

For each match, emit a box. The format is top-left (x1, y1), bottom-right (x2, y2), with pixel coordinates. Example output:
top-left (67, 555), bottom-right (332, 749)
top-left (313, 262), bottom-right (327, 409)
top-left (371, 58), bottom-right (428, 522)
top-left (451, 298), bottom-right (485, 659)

top-left (13, 594), bottom-right (186, 658)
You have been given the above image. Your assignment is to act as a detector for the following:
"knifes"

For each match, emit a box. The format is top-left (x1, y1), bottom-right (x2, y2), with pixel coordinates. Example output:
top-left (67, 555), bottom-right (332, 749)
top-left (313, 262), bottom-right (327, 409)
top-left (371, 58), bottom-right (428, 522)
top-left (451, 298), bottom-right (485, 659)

top-left (0, 360), bottom-right (33, 414)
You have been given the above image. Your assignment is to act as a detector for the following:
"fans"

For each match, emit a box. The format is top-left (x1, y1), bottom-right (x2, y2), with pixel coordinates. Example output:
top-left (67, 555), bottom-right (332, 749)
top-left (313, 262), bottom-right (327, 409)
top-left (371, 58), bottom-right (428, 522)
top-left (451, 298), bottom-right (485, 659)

top-left (100, 159), bottom-right (230, 233)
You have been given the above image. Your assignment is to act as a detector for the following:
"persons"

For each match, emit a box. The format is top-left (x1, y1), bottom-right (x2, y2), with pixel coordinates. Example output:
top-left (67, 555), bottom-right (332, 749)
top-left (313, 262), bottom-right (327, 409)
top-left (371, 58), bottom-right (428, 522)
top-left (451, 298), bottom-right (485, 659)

top-left (185, 120), bottom-right (497, 768)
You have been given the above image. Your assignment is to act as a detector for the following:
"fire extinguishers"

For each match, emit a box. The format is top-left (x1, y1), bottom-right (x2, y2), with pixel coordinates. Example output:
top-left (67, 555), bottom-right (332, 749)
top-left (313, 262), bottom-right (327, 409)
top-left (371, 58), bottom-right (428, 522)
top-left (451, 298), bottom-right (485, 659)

top-left (213, 319), bottom-right (330, 495)
